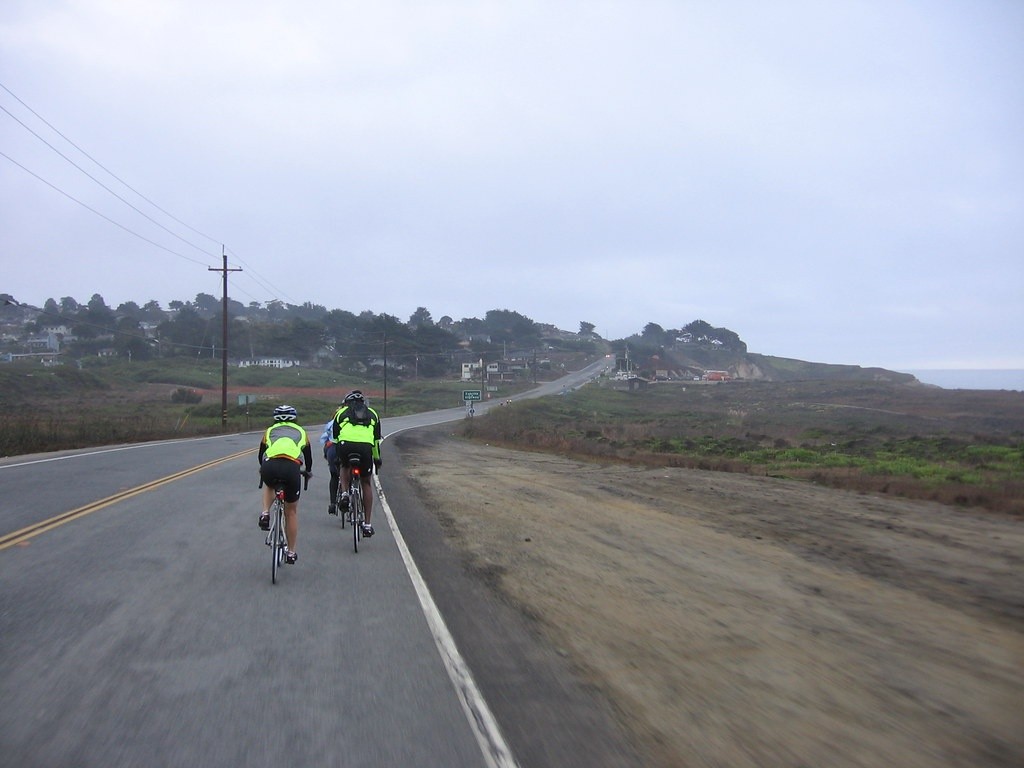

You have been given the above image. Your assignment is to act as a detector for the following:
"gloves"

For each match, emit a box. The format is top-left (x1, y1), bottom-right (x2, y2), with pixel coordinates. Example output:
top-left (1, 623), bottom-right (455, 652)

top-left (373, 458), bottom-right (384, 470)
top-left (302, 469), bottom-right (312, 480)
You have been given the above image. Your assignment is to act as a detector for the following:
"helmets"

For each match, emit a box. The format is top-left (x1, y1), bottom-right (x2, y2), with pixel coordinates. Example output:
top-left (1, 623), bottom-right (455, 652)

top-left (273, 405), bottom-right (297, 421)
top-left (342, 390), bottom-right (370, 407)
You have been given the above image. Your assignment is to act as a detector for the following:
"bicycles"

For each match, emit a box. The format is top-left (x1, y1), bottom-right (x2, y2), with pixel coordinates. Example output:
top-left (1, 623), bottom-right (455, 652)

top-left (259, 470), bottom-right (310, 585)
top-left (326, 452), bottom-right (380, 554)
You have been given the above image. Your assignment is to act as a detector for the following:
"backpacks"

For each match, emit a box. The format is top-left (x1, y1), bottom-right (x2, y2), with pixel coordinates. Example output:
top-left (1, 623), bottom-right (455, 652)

top-left (339, 400), bottom-right (378, 428)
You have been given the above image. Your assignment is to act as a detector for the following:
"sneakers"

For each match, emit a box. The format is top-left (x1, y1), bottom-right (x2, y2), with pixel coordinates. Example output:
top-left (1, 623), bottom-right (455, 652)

top-left (338, 492), bottom-right (350, 513)
top-left (362, 522), bottom-right (376, 537)
top-left (257, 513), bottom-right (270, 529)
top-left (287, 551), bottom-right (298, 564)
top-left (327, 504), bottom-right (339, 517)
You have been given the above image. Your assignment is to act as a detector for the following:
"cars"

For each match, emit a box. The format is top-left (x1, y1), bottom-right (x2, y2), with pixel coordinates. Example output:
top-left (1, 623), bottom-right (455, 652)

top-left (653, 375), bottom-right (671, 383)
top-left (693, 376), bottom-right (700, 381)
top-left (703, 375), bottom-right (707, 380)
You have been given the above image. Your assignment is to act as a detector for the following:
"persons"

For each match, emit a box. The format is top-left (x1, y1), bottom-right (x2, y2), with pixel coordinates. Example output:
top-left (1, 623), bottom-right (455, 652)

top-left (258, 404), bottom-right (313, 564)
top-left (320, 418), bottom-right (341, 515)
top-left (333, 389), bottom-right (382, 538)
